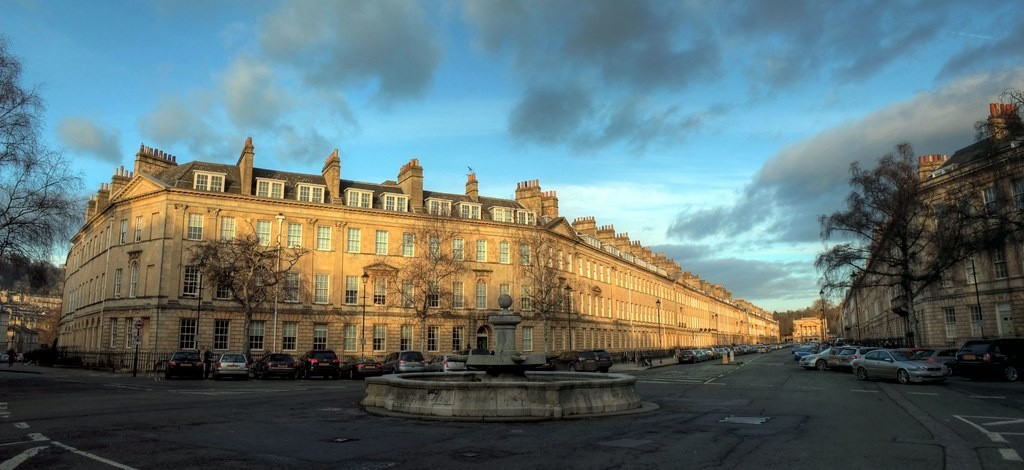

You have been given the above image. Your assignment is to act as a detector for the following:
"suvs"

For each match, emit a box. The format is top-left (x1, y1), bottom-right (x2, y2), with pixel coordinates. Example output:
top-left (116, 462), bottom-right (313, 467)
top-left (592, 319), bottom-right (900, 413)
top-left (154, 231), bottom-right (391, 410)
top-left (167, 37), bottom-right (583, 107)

top-left (549, 349), bottom-right (613, 372)
top-left (827, 346), bottom-right (877, 373)
top-left (165, 351), bottom-right (204, 378)
top-left (298, 349), bottom-right (340, 379)
top-left (954, 336), bottom-right (1024, 381)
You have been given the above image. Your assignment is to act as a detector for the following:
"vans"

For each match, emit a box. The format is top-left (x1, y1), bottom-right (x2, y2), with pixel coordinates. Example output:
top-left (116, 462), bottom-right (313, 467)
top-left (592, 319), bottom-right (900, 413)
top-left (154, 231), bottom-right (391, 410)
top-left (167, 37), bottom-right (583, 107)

top-left (426, 348), bottom-right (490, 372)
top-left (253, 354), bottom-right (297, 379)
top-left (381, 351), bottom-right (425, 375)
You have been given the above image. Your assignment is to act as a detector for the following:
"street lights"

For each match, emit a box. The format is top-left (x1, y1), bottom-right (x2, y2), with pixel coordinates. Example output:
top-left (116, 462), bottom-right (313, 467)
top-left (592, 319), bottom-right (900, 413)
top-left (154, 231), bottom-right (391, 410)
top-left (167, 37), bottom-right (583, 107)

top-left (656, 300), bottom-right (663, 364)
top-left (361, 271), bottom-right (370, 358)
top-left (820, 290), bottom-right (826, 341)
top-left (196, 260), bottom-right (206, 349)
top-left (850, 271), bottom-right (861, 346)
top-left (564, 284), bottom-right (572, 350)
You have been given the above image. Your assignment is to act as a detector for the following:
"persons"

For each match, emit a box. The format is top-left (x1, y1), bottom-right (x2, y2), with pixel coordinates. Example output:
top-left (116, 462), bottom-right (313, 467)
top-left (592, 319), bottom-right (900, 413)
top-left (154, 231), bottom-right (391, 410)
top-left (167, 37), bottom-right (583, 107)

top-left (8, 348), bottom-right (17, 367)
top-left (883, 339), bottom-right (892, 348)
top-left (246, 348), bottom-right (252, 365)
top-left (203, 348), bottom-right (214, 379)
top-left (621, 350), bottom-right (648, 367)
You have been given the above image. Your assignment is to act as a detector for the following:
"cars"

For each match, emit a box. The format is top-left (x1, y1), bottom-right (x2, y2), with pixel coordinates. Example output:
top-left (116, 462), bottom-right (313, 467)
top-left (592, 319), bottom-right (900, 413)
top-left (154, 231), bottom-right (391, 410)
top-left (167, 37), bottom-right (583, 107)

top-left (341, 357), bottom-right (382, 380)
top-left (914, 347), bottom-right (961, 377)
top-left (679, 342), bottom-right (792, 364)
top-left (853, 349), bottom-right (947, 384)
top-left (792, 342), bottom-right (844, 371)
top-left (212, 352), bottom-right (250, 378)
